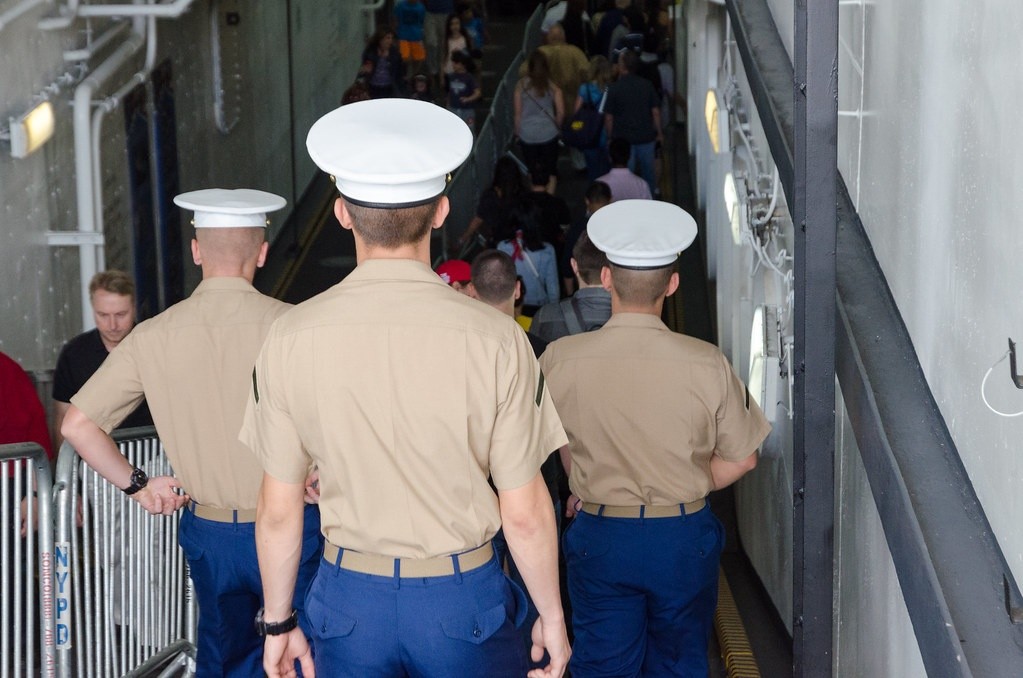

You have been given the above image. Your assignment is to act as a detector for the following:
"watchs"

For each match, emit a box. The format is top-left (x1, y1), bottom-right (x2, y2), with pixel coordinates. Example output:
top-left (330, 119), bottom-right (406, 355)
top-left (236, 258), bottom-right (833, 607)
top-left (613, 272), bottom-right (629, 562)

top-left (120, 467), bottom-right (148, 495)
top-left (254, 607), bottom-right (298, 639)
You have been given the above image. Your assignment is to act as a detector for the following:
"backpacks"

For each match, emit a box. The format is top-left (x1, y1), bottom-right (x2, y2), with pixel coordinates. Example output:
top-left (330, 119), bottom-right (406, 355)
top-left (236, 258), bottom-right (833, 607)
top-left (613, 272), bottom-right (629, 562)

top-left (643, 62), bottom-right (664, 103)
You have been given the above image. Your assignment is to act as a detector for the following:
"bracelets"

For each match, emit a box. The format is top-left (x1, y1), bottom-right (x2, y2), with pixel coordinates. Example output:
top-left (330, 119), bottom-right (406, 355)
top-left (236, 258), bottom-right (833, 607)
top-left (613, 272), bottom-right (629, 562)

top-left (33, 491), bottom-right (37, 498)
top-left (458, 237), bottom-right (464, 244)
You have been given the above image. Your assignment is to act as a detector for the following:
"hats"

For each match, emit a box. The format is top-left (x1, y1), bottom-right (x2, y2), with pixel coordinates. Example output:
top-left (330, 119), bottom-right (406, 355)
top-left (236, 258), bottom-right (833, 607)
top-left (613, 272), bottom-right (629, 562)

top-left (586, 198), bottom-right (698, 269)
top-left (306, 98), bottom-right (474, 208)
top-left (414, 60), bottom-right (433, 81)
top-left (172, 188), bottom-right (286, 228)
top-left (434, 259), bottom-right (473, 285)
top-left (451, 47), bottom-right (475, 73)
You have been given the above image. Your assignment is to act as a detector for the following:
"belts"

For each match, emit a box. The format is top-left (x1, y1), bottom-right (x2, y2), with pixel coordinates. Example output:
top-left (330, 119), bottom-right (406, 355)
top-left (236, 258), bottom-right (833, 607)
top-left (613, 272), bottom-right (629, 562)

top-left (185, 500), bottom-right (308, 523)
top-left (581, 498), bottom-right (706, 518)
top-left (323, 538), bottom-right (494, 579)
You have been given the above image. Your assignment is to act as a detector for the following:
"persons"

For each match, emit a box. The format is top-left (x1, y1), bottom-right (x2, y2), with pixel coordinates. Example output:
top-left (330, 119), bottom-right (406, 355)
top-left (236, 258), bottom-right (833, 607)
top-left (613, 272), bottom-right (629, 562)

top-left (536, 199), bottom-right (773, 678)
top-left (60, 188), bottom-right (325, 678)
top-left (0, 350), bottom-right (53, 678)
top-left (341, 0), bottom-right (492, 140)
top-left (513, 0), bottom-right (675, 200)
top-left (449, 156), bottom-right (612, 300)
top-left (236, 98), bottom-right (573, 678)
top-left (52, 269), bottom-right (179, 655)
top-left (435, 230), bottom-right (612, 678)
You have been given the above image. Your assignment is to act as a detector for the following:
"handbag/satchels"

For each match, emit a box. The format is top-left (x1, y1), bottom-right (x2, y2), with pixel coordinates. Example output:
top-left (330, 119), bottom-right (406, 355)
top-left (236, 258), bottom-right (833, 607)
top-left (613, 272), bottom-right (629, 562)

top-left (562, 102), bottom-right (605, 150)
top-left (554, 135), bottom-right (570, 157)
